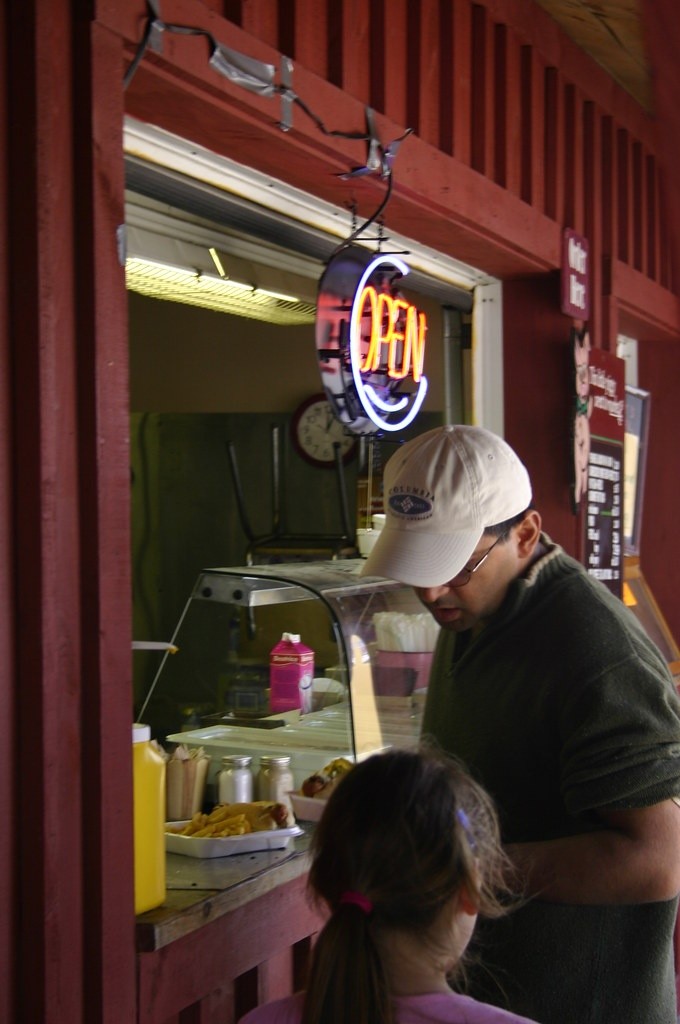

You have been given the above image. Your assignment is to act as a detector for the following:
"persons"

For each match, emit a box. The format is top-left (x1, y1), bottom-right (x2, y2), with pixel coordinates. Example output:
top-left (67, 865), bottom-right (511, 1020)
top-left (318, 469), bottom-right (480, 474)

top-left (234, 748), bottom-right (538, 1024)
top-left (360, 424), bottom-right (680, 1024)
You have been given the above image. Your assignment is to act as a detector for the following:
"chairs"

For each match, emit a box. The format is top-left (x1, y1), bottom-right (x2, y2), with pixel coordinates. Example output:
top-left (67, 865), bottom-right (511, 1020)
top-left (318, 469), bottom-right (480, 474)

top-left (224, 425), bottom-right (358, 667)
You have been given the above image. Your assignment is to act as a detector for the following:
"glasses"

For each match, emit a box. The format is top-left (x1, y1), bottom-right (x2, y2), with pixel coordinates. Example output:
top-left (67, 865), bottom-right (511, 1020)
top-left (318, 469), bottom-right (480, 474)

top-left (441, 534), bottom-right (506, 588)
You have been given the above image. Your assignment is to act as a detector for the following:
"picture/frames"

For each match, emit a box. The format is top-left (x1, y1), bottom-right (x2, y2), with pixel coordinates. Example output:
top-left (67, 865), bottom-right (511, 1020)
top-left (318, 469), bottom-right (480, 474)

top-left (622, 384), bottom-right (653, 555)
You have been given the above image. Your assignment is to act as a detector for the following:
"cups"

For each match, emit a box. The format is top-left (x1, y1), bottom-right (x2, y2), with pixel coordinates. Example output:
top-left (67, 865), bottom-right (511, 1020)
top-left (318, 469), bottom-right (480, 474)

top-left (167, 755), bottom-right (211, 822)
top-left (372, 514), bottom-right (386, 529)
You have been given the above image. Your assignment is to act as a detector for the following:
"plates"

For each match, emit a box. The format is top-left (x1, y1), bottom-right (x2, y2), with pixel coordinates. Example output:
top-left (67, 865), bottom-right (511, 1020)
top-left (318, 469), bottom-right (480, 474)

top-left (165, 821), bottom-right (305, 859)
top-left (288, 791), bottom-right (327, 822)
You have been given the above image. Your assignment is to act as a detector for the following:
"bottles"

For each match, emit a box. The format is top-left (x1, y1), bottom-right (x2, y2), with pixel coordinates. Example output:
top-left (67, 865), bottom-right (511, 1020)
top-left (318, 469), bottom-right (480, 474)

top-left (132, 722), bottom-right (167, 916)
top-left (217, 754), bottom-right (253, 806)
top-left (256, 755), bottom-right (295, 812)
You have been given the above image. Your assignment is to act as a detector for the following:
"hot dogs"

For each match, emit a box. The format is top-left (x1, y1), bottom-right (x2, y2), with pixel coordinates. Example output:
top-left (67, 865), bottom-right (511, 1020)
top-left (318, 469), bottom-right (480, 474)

top-left (212, 800), bottom-right (292, 829)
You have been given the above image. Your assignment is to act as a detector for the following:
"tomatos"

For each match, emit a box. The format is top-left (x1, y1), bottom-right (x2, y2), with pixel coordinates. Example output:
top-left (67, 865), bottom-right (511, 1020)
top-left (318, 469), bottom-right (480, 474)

top-left (302, 776), bottom-right (326, 797)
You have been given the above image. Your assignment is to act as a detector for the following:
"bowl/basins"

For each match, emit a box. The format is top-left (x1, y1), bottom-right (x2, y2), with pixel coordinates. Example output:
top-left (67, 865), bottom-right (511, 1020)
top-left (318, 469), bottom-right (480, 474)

top-left (357, 528), bottom-right (382, 558)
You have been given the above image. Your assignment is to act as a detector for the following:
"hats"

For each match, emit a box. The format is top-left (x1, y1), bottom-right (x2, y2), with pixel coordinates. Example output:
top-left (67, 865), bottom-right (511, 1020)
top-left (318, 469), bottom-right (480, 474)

top-left (359, 424), bottom-right (533, 588)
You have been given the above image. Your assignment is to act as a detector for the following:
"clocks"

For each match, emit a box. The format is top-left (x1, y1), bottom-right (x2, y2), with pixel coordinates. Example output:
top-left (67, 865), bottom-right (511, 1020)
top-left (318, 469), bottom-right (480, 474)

top-left (289, 393), bottom-right (358, 469)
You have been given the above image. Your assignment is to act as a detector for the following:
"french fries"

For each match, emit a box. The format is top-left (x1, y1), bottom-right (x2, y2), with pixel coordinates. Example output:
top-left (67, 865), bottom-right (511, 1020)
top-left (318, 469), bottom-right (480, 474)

top-left (175, 812), bottom-right (250, 839)
